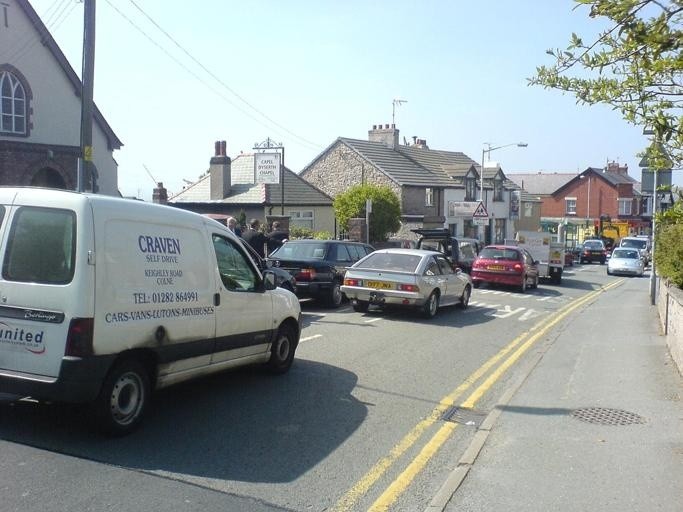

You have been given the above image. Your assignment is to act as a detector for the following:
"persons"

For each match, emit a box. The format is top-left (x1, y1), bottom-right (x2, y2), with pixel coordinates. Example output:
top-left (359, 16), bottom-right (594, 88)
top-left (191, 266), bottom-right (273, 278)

top-left (225, 217), bottom-right (241, 239)
top-left (239, 218), bottom-right (288, 273)
top-left (266, 220), bottom-right (288, 258)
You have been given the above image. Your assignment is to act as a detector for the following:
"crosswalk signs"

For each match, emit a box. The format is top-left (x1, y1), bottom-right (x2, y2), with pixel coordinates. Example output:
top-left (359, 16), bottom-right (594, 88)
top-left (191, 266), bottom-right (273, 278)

top-left (472, 201), bottom-right (488, 217)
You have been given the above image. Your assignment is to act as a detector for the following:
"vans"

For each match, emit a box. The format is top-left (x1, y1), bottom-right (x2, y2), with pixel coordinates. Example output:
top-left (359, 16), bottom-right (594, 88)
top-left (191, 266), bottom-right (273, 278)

top-left (0, 184), bottom-right (302, 437)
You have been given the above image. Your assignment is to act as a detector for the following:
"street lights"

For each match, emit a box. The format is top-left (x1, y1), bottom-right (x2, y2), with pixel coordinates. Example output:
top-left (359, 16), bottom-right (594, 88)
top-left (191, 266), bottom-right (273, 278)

top-left (478, 140), bottom-right (528, 245)
top-left (642, 129), bottom-right (661, 305)
top-left (580, 175), bottom-right (590, 218)
